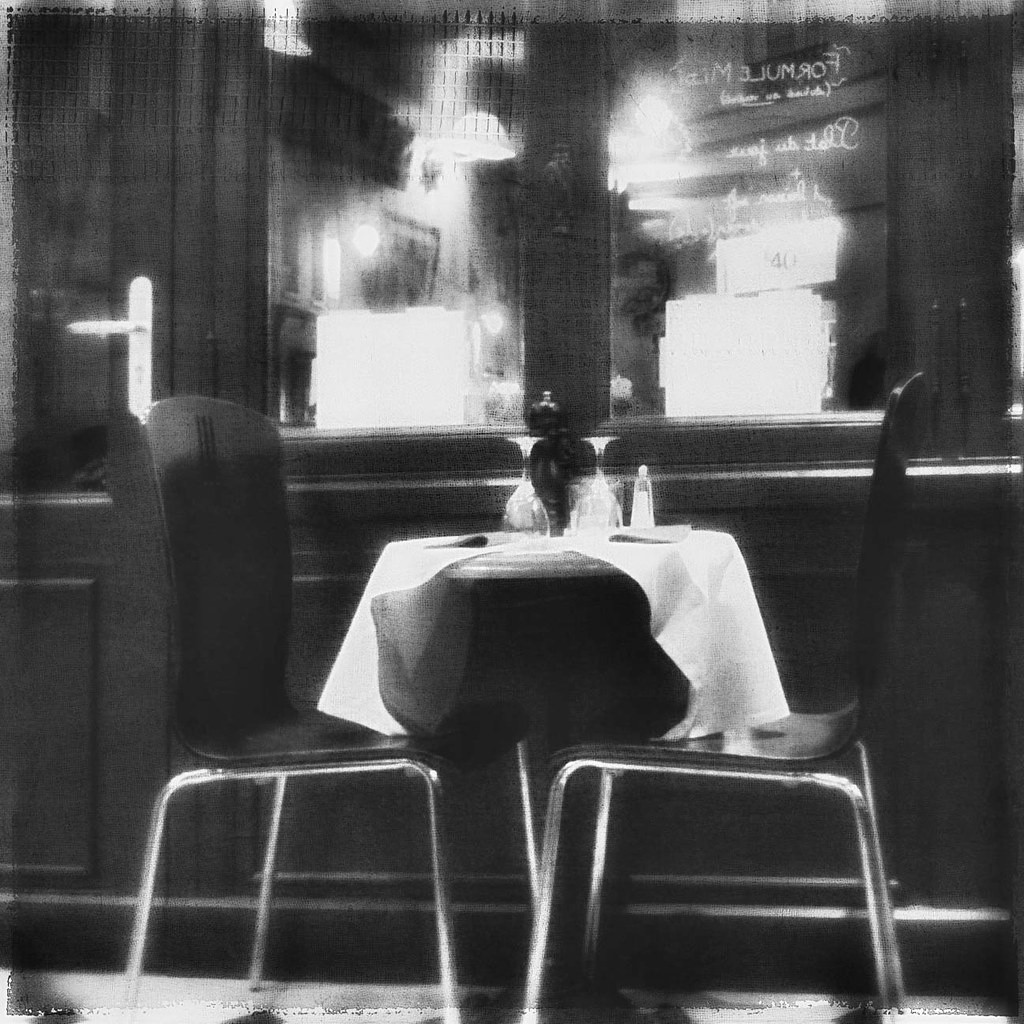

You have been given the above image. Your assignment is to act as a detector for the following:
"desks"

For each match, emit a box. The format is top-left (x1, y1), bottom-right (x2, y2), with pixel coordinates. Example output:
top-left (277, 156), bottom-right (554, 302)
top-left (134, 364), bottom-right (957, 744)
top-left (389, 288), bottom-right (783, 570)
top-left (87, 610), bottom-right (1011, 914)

top-left (314, 527), bottom-right (791, 1024)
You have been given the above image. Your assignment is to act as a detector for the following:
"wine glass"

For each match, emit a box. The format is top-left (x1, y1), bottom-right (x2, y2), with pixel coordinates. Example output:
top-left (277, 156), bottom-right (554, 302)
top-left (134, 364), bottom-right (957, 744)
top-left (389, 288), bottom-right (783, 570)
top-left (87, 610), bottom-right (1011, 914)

top-left (575, 437), bottom-right (623, 534)
top-left (501, 437), bottom-right (551, 546)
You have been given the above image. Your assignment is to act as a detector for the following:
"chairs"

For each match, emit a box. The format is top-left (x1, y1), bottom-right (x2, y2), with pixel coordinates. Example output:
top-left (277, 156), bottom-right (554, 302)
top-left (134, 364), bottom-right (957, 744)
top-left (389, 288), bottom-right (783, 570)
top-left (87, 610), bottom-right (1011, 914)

top-left (125, 394), bottom-right (459, 1012)
top-left (524, 370), bottom-right (936, 1012)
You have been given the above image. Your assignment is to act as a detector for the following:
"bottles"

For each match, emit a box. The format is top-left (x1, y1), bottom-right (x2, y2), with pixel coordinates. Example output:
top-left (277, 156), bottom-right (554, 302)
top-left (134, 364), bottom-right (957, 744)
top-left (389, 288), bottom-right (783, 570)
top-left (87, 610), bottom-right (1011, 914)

top-left (631, 464), bottom-right (655, 529)
